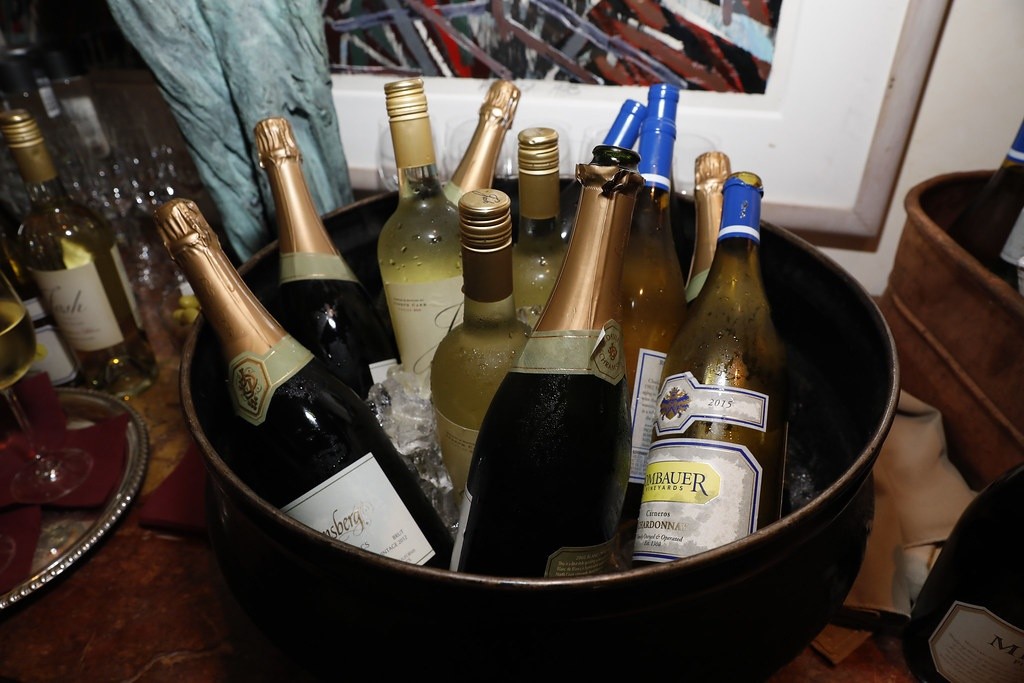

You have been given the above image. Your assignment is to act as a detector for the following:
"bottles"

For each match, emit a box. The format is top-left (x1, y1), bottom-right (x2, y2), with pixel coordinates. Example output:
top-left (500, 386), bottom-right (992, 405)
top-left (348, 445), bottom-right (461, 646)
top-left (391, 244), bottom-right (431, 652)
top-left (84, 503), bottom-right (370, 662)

top-left (451, 144), bottom-right (644, 580)
top-left (901, 461), bottom-right (1024, 683)
top-left (0, 44), bottom-right (161, 403)
top-left (1002, 119), bottom-right (1024, 177)
top-left (512, 130), bottom-right (570, 332)
top-left (560, 101), bottom-right (647, 245)
top-left (447, 78), bottom-right (521, 190)
top-left (621, 119), bottom-right (685, 499)
top-left (646, 84), bottom-right (673, 204)
top-left (686, 153), bottom-right (732, 314)
top-left (252, 115), bottom-right (400, 398)
top-left (155, 199), bottom-right (456, 572)
top-left (377, 80), bottom-right (463, 375)
top-left (632, 172), bottom-right (789, 572)
top-left (431, 190), bottom-right (533, 496)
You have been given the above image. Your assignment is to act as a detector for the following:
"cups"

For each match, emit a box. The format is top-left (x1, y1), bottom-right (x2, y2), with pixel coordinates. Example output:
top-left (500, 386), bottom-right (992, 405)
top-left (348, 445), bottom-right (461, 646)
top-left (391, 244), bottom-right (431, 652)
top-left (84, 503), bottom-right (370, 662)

top-left (0, 97), bottom-right (192, 298)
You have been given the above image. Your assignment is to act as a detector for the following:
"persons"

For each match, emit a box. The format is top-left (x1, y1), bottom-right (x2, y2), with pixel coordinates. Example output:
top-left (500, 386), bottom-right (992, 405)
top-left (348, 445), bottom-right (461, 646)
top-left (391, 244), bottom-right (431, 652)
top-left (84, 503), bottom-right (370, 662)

top-left (106, 0), bottom-right (355, 264)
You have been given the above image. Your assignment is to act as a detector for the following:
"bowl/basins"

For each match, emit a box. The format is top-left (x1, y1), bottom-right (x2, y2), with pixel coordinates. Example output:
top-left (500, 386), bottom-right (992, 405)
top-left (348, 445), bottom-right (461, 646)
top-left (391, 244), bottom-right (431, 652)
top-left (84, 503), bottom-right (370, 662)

top-left (182, 173), bottom-right (898, 683)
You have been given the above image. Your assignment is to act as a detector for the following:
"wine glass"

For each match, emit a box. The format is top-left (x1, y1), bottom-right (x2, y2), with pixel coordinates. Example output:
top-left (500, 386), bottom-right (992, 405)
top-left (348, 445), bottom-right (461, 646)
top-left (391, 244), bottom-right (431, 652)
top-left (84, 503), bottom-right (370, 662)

top-left (0, 269), bottom-right (92, 503)
